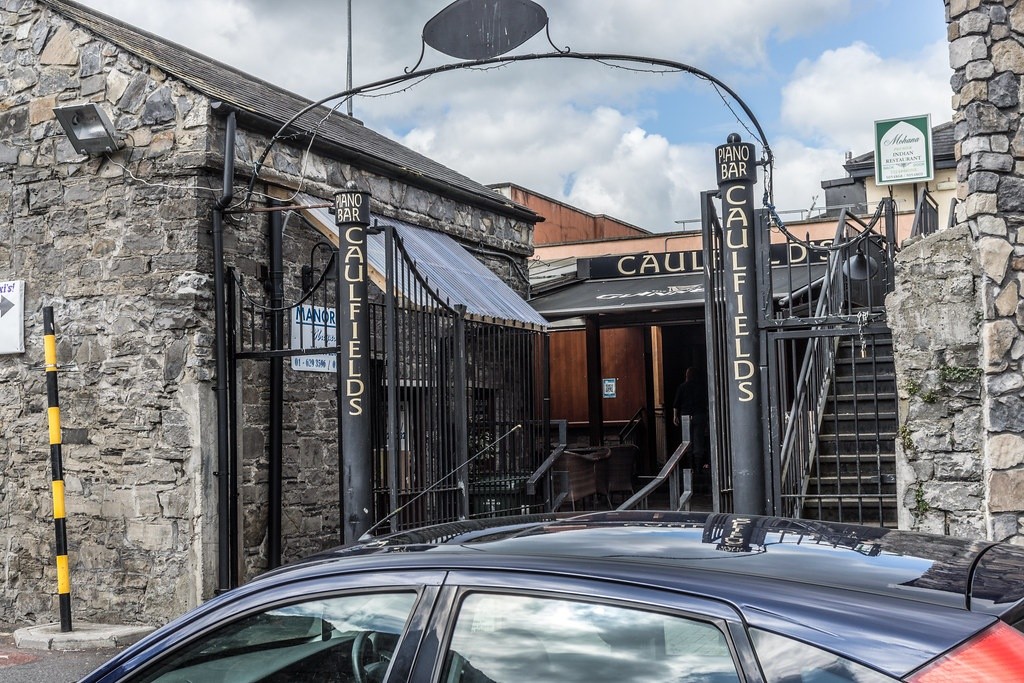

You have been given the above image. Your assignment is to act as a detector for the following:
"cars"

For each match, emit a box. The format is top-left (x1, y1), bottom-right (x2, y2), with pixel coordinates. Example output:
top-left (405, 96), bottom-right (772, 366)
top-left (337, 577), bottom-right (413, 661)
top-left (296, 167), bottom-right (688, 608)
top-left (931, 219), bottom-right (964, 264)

top-left (73, 509), bottom-right (1024, 683)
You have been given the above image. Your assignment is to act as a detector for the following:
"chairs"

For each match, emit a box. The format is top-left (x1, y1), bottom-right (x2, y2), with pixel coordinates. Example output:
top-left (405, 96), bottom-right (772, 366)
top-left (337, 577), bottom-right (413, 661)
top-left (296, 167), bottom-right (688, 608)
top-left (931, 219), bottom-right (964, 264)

top-left (548, 448), bottom-right (612, 512)
top-left (608, 444), bottom-right (639, 509)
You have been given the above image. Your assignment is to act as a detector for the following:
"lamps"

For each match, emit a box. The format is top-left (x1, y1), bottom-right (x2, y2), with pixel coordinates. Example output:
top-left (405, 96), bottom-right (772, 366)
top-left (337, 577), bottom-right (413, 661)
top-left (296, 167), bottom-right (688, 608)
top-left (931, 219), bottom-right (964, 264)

top-left (52, 104), bottom-right (124, 160)
top-left (302, 241), bottom-right (337, 293)
top-left (843, 234), bottom-right (886, 281)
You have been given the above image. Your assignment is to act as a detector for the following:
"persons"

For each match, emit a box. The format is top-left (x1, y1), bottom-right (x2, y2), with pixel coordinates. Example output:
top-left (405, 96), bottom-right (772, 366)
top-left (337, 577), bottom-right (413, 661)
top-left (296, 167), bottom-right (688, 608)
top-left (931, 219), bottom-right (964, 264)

top-left (673, 366), bottom-right (707, 473)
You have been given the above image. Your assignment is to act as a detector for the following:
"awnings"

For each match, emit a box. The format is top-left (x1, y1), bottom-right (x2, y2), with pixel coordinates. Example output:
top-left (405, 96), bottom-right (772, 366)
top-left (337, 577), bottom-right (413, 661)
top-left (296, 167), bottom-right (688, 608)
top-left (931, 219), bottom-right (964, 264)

top-left (526, 260), bottom-right (844, 316)
top-left (293, 194), bottom-right (550, 334)
